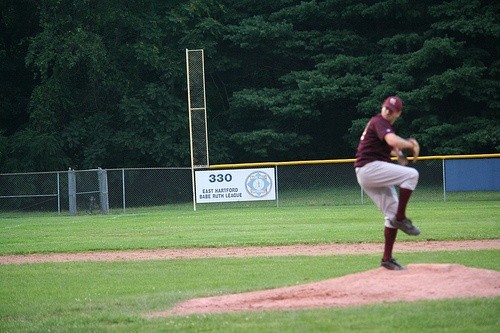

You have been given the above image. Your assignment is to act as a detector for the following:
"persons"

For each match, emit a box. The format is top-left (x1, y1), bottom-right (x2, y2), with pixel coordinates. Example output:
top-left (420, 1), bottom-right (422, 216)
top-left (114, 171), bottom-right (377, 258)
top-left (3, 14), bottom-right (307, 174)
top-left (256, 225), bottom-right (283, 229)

top-left (354, 97), bottom-right (420, 271)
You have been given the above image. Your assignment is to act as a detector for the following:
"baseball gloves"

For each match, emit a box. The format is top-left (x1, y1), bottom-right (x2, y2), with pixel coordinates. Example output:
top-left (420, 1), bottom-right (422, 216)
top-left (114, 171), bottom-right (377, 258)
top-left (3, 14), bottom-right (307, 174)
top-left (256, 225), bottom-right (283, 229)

top-left (409, 137), bottom-right (419, 164)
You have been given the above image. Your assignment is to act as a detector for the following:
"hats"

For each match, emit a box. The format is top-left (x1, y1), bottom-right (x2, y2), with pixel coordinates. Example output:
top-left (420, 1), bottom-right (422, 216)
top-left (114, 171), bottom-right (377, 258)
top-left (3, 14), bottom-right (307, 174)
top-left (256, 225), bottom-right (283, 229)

top-left (383, 95), bottom-right (402, 113)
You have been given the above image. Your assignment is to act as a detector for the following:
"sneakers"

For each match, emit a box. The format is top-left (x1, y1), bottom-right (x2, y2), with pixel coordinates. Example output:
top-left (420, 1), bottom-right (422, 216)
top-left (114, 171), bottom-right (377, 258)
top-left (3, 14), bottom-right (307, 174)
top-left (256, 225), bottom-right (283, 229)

top-left (389, 213), bottom-right (421, 237)
top-left (380, 256), bottom-right (403, 272)
